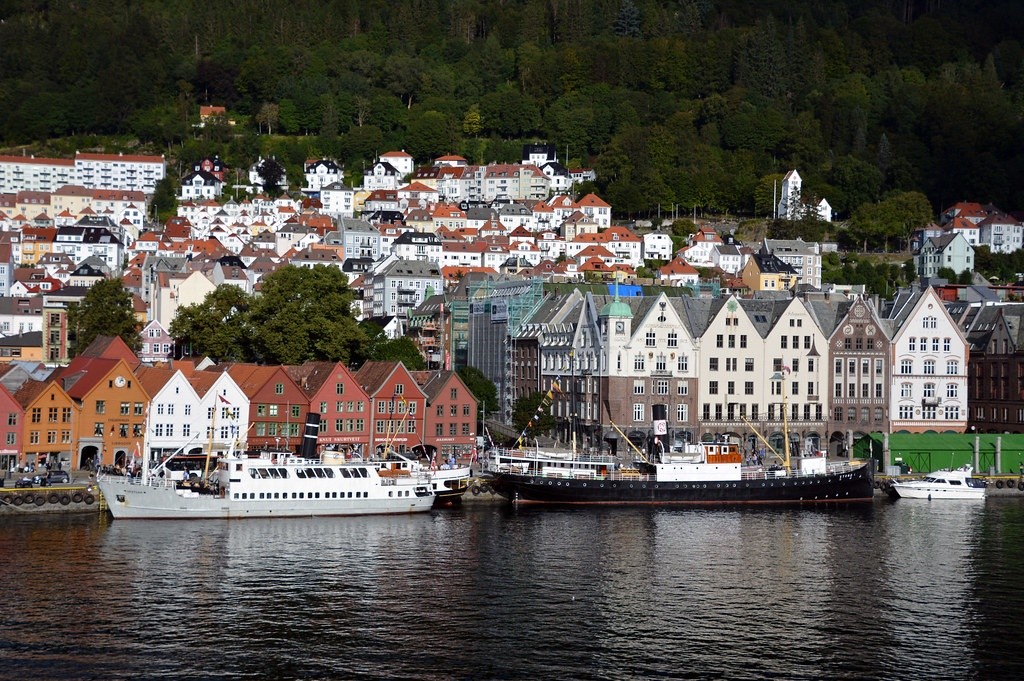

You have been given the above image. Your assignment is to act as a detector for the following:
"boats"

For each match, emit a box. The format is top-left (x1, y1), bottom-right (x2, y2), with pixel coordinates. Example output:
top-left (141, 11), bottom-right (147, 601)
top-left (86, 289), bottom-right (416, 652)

top-left (468, 347), bottom-right (879, 508)
top-left (96, 389), bottom-right (472, 519)
top-left (890, 451), bottom-right (991, 500)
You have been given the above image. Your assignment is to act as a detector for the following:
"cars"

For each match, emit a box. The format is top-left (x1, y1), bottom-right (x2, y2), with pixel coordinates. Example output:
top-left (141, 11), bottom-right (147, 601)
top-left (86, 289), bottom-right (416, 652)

top-left (15, 477), bottom-right (33, 489)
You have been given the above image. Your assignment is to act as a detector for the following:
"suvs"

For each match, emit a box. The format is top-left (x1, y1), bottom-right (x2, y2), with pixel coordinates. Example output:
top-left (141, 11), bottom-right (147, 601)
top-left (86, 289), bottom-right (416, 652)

top-left (33, 470), bottom-right (70, 485)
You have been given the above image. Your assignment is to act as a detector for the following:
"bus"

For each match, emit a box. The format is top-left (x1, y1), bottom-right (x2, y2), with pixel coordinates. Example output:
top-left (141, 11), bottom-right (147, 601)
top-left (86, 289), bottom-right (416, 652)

top-left (162, 453), bottom-right (276, 484)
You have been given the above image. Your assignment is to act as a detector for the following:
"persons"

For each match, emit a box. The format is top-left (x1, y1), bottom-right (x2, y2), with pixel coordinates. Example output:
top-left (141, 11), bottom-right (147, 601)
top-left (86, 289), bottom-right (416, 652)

top-left (23, 458), bottom-right (62, 473)
top-left (101, 463), bottom-right (142, 477)
top-left (183, 466), bottom-right (190, 483)
top-left (773, 460), bottom-right (778, 471)
top-left (745, 448), bottom-right (765, 466)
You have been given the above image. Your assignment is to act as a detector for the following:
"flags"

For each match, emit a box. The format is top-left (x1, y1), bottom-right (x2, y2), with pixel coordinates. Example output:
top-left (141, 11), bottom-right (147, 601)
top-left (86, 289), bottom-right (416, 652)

top-left (518, 380), bottom-right (566, 445)
top-left (219, 394), bottom-right (232, 405)
